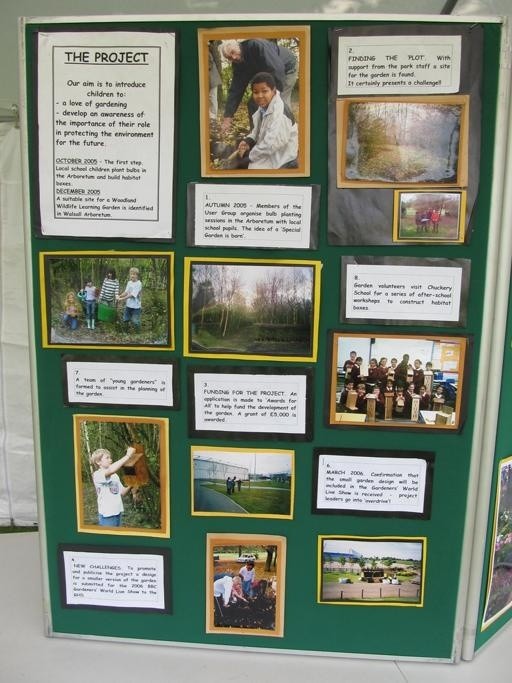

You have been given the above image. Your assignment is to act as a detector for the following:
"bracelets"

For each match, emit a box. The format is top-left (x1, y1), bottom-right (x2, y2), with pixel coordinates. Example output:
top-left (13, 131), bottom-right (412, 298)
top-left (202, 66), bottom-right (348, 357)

top-left (126, 453), bottom-right (132, 459)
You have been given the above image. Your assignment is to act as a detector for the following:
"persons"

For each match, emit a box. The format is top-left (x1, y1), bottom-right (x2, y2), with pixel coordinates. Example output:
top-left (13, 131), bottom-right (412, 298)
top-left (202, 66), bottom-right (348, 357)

top-left (208, 51), bottom-right (222, 119)
top-left (236, 477), bottom-right (244, 491)
top-left (83, 278), bottom-right (99, 328)
top-left (220, 71), bottom-right (299, 169)
top-left (90, 446), bottom-right (137, 528)
top-left (117, 266), bottom-right (142, 333)
top-left (226, 476), bottom-right (232, 494)
top-left (214, 559), bottom-right (276, 631)
top-left (62, 290), bottom-right (81, 329)
top-left (207, 39), bottom-right (225, 109)
top-left (218, 38), bottom-right (297, 132)
top-left (231, 475), bottom-right (236, 492)
top-left (415, 205), bottom-right (439, 232)
top-left (339, 350), bottom-right (445, 419)
top-left (97, 266), bottom-right (120, 306)
